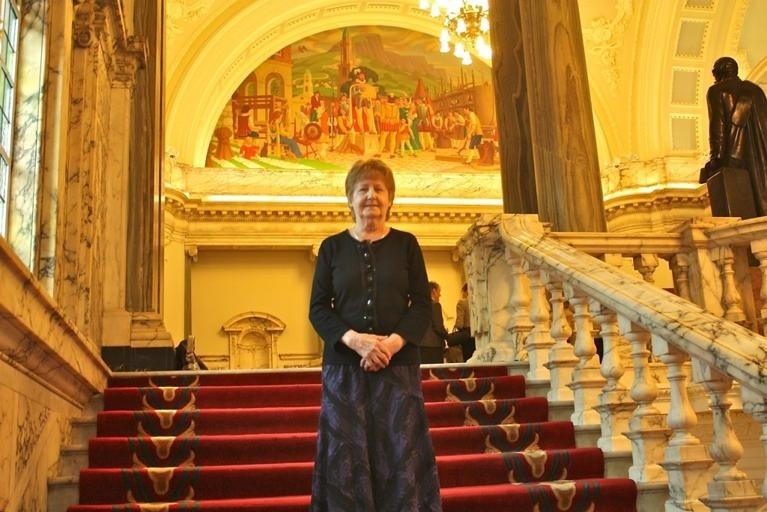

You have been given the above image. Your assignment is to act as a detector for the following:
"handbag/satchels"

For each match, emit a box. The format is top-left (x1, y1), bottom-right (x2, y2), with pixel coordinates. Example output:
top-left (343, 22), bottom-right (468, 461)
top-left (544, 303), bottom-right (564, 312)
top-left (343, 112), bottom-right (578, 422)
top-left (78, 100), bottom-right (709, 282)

top-left (446, 330), bottom-right (469, 346)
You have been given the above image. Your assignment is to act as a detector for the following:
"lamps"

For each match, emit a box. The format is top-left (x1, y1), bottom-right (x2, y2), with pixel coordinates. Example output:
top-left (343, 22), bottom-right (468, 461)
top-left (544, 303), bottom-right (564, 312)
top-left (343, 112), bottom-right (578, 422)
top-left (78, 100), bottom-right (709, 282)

top-left (420, 0), bottom-right (493, 64)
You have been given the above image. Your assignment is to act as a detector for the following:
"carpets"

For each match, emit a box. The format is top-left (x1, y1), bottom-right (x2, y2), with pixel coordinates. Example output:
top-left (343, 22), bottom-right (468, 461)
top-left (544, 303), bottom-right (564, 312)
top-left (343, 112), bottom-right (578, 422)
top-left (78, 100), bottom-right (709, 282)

top-left (65, 365), bottom-right (638, 511)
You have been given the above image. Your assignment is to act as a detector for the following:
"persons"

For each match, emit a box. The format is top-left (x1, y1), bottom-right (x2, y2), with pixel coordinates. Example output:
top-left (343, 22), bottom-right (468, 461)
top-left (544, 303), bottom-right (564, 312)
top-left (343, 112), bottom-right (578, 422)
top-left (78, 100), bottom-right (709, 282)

top-left (418, 282), bottom-right (448, 364)
top-left (456, 283), bottom-right (476, 362)
top-left (214, 69), bottom-right (482, 165)
top-left (311, 160), bottom-right (444, 512)
top-left (705, 57), bottom-right (767, 217)
